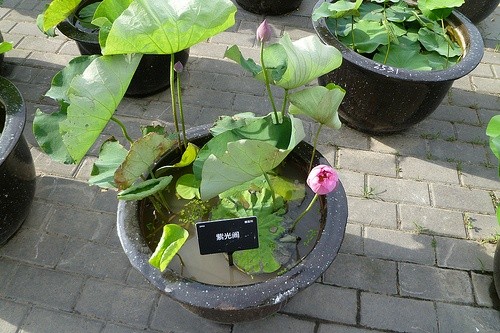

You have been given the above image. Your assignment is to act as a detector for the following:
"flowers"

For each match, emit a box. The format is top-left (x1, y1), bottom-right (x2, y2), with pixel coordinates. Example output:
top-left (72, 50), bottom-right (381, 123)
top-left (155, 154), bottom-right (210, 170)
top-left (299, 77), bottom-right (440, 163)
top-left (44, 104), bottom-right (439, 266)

top-left (29, 1), bottom-right (346, 283)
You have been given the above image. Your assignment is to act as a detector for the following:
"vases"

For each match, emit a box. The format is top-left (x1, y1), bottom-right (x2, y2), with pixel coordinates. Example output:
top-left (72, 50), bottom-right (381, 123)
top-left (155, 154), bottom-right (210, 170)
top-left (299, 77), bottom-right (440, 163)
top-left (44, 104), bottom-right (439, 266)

top-left (114, 122), bottom-right (350, 325)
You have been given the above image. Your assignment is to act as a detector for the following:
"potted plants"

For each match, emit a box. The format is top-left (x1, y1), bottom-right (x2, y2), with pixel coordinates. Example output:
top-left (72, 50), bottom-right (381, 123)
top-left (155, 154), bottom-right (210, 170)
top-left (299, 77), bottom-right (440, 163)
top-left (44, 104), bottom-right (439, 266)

top-left (40, 0), bottom-right (190, 99)
top-left (309, 0), bottom-right (488, 135)
top-left (0, 39), bottom-right (37, 251)
